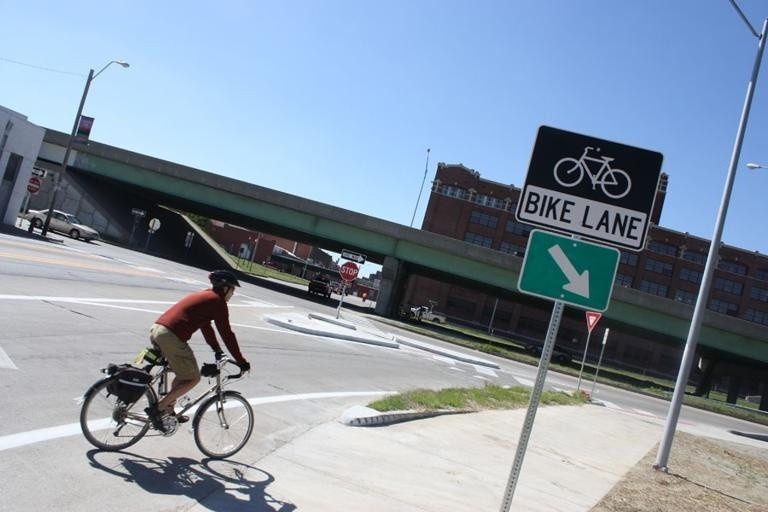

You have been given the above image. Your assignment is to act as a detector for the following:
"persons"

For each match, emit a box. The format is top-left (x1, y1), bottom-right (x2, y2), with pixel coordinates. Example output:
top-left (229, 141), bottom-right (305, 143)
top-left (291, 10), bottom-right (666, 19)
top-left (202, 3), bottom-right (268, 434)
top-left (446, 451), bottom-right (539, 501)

top-left (144, 270), bottom-right (250, 433)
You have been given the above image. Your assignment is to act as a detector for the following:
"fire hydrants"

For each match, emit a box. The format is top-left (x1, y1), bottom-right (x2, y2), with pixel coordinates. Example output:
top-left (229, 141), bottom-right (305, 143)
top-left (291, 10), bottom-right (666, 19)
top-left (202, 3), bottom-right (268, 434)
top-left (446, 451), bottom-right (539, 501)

top-left (28, 217), bottom-right (37, 233)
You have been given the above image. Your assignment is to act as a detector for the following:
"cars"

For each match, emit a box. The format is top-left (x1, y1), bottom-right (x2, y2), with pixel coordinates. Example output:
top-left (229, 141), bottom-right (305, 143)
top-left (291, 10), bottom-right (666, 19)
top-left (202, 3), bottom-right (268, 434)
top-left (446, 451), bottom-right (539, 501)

top-left (308, 275), bottom-right (349, 299)
top-left (26, 208), bottom-right (99, 241)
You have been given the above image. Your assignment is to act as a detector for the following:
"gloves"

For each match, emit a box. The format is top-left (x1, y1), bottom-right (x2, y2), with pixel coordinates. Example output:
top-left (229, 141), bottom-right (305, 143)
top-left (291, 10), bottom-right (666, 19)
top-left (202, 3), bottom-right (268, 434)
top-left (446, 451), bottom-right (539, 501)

top-left (215, 351), bottom-right (223, 360)
top-left (240, 362), bottom-right (249, 371)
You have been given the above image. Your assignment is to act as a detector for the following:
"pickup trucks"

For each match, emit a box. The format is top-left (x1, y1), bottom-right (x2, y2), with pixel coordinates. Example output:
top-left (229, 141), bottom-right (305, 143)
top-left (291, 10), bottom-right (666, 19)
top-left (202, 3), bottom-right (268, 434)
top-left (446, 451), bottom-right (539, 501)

top-left (410, 306), bottom-right (446, 324)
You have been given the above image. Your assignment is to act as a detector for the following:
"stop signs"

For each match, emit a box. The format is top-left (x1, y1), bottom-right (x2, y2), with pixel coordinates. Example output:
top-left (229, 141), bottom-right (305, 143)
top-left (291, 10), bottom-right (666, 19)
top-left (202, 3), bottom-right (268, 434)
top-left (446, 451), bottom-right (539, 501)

top-left (339, 262), bottom-right (358, 282)
top-left (26, 177), bottom-right (40, 193)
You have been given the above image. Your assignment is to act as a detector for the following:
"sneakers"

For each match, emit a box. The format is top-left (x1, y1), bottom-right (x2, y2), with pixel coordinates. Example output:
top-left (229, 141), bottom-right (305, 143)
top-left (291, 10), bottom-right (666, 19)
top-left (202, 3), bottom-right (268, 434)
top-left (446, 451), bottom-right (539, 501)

top-left (144, 406), bottom-right (164, 430)
top-left (171, 411), bottom-right (189, 423)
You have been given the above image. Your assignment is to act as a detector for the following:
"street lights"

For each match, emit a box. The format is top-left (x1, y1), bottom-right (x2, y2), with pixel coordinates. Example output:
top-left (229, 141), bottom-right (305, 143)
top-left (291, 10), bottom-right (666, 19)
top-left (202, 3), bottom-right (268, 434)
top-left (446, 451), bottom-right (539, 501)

top-left (748, 163), bottom-right (763, 172)
top-left (41, 59), bottom-right (129, 236)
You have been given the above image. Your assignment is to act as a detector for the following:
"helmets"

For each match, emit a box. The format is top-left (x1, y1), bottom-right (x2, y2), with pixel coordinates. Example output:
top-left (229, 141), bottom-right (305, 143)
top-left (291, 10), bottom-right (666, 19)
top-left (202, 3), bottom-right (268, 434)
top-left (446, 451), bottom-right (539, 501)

top-left (209, 270), bottom-right (241, 289)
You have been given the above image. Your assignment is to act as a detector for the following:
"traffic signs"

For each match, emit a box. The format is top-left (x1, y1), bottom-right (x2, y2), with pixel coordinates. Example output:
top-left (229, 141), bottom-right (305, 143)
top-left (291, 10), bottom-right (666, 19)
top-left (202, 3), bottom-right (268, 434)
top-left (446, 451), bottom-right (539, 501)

top-left (341, 249), bottom-right (366, 264)
top-left (31, 167), bottom-right (46, 177)
top-left (131, 208), bottom-right (147, 217)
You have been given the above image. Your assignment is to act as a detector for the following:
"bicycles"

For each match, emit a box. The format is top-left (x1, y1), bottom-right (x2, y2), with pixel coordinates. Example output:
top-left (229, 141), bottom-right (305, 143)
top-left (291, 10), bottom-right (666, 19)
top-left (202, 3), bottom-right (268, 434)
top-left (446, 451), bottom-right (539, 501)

top-left (79, 346), bottom-right (253, 458)
